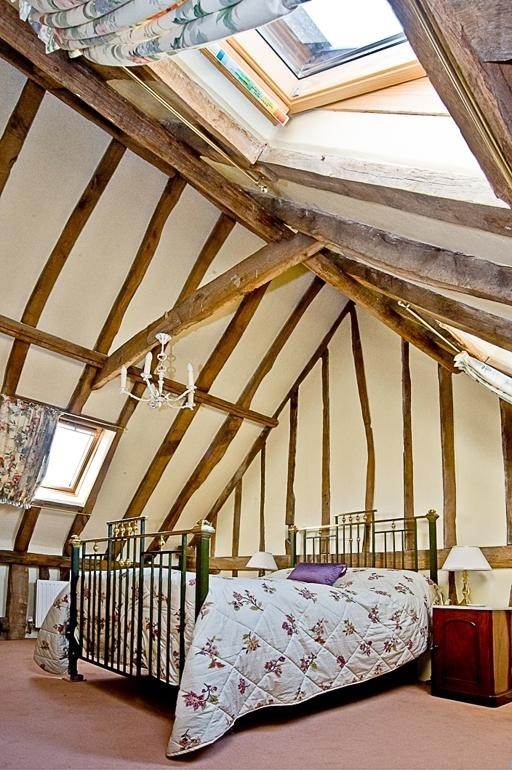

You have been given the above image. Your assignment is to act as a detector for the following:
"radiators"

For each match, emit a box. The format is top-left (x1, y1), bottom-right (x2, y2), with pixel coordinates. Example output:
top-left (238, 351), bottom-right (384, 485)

top-left (27, 579), bottom-right (70, 634)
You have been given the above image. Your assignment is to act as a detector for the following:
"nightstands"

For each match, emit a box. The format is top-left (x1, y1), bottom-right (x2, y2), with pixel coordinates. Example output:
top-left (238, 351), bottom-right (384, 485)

top-left (432, 605), bottom-right (512, 708)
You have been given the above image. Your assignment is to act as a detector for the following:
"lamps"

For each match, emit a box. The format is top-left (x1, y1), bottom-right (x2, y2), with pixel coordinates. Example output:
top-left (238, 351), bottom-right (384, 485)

top-left (245, 552), bottom-right (279, 577)
top-left (119, 333), bottom-right (197, 411)
top-left (441, 546), bottom-right (493, 607)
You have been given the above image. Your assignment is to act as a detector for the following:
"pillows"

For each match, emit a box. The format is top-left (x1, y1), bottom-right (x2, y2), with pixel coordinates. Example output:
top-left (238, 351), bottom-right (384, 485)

top-left (287, 563), bottom-right (347, 585)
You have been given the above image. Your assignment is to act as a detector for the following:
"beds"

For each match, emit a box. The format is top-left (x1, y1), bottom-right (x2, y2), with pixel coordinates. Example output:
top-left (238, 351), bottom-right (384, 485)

top-left (68, 508), bottom-right (440, 691)
